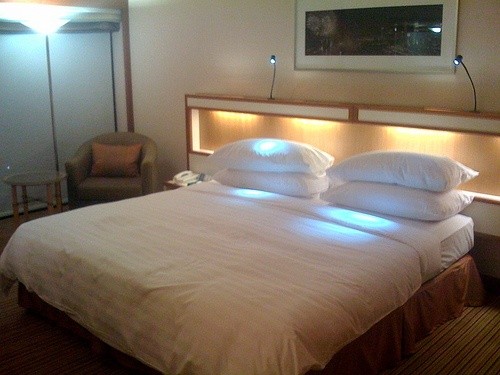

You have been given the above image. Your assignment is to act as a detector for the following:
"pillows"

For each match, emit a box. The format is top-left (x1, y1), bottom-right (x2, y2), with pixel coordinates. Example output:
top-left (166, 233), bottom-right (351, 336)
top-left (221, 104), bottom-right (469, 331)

top-left (208, 168), bottom-right (331, 197)
top-left (325, 151), bottom-right (479, 192)
top-left (92, 140), bottom-right (141, 176)
top-left (319, 182), bottom-right (475, 220)
top-left (205, 138), bottom-right (334, 179)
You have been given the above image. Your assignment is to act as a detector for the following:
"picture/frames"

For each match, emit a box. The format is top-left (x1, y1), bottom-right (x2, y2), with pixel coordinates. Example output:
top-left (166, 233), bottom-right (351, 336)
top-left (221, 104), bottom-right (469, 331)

top-left (294, 1), bottom-right (459, 75)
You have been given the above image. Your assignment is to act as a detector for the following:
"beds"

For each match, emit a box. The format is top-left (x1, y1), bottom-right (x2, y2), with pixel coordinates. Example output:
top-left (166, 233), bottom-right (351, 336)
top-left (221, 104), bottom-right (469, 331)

top-left (0, 94), bottom-right (482, 373)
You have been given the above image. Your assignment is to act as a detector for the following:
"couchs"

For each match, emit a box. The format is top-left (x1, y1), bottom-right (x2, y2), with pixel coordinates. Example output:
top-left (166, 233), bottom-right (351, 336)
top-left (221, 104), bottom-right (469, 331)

top-left (66, 132), bottom-right (158, 208)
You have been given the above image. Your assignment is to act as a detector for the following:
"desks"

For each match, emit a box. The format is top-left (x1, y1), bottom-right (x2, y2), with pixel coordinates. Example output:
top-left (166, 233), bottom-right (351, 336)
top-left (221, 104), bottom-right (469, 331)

top-left (5, 170), bottom-right (66, 225)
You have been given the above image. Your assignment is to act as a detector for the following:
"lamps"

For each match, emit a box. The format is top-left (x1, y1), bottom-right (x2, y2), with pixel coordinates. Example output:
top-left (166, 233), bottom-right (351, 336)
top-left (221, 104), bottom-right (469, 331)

top-left (452, 53), bottom-right (465, 67)
top-left (268, 54), bottom-right (280, 68)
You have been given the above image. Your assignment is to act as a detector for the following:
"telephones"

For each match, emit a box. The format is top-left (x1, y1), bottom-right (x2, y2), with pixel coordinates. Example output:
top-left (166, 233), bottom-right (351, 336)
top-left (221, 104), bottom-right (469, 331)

top-left (173, 170), bottom-right (198, 183)
